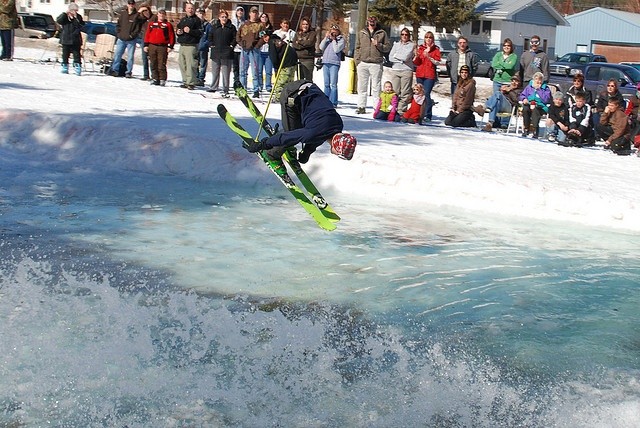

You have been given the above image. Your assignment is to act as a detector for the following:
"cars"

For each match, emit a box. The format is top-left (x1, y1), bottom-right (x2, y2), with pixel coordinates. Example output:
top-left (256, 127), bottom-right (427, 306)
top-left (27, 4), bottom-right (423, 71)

top-left (436, 50), bottom-right (493, 77)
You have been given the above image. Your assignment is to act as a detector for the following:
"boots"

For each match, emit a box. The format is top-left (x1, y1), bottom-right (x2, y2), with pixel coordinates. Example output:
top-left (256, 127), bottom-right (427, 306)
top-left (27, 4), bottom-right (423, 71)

top-left (74, 63), bottom-right (81, 75)
top-left (62, 64), bottom-right (68, 73)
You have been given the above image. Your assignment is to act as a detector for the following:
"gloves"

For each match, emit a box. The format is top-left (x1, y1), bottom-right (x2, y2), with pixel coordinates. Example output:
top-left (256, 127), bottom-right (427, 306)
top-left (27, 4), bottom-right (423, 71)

top-left (242, 137), bottom-right (273, 153)
top-left (299, 144), bottom-right (316, 163)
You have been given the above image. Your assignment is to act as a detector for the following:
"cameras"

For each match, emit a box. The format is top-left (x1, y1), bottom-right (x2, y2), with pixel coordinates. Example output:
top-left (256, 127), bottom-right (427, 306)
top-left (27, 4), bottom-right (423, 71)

top-left (331, 32), bottom-right (336, 37)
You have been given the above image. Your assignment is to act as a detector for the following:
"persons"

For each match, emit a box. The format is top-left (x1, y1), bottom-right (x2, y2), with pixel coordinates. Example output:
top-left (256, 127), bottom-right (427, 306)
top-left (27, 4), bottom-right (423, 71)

top-left (519, 34), bottom-right (550, 94)
top-left (351, 12), bottom-right (391, 114)
top-left (176, 2), bottom-right (204, 90)
top-left (490, 38), bottom-right (518, 96)
top-left (624, 81), bottom-right (640, 142)
top-left (272, 17), bottom-right (297, 48)
top-left (256, 11), bottom-right (275, 93)
top-left (231, 5), bottom-right (247, 94)
top-left (558, 92), bottom-right (596, 148)
top-left (469, 71), bottom-right (524, 132)
top-left (0, 0), bottom-right (19, 61)
top-left (399, 83), bottom-right (426, 126)
top-left (205, 9), bottom-right (237, 98)
top-left (444, 65), bottom-right (478, 128)
top-left (516, 71), bottom-right (553, 140)
top-left (543, 91), bottom-right (571, 145)
top-left (591, 78), bottom-right (627, 142)
top-left (595, 97), bottom-right (633, 155)
top-left (318, 24), bottom-right (345, 108)
top-left (564, 72), bottom-right (592, 123)
top-left (292, 16), bottom-right (317, 83)
top-left (57, 2), bottom-right (86, 76)
top-left (193, 7), bottom-right (213, 86)
top-left (142, 9), bottom-right (175, 86)
top-left (445, 36), bottom-right (479, 110)
top-left (411, 31), bottom-right (441, 123)
top-left (235, 6), bottom-right (268, 98)
top-left (388, 27), bottom-right (417, 117)
top-left (243, 80), bottom-right (357, 178)
top-left (109, 0), bottom-right (142, 77)
top-left (373, 81), bottom-right (404, 122)
top-left (258, 30), bottom-right (298, 103)
top-left (136, 3), bottom-right (157, 81)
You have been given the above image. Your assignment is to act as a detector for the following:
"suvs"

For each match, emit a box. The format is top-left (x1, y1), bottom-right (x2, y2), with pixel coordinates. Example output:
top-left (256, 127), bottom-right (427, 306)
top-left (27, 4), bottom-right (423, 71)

top-left (14, 15), bottom-right (56, 39)
top-left (551, 53), bottom-right (606, 75)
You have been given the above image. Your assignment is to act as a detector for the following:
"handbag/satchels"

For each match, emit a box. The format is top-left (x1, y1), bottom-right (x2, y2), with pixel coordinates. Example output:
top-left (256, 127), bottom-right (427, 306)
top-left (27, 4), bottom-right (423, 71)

top-left (486, 67), bottom-right (495, 81)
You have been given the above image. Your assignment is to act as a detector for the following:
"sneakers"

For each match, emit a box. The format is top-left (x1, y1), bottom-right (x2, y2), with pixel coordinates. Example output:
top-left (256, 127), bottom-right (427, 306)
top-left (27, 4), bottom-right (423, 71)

top-left (150, 80), bottom-right (160, 85)
top-left (253, 92), bottom-right (259, 98)
top-left (285, 146), bottom-right (300, 169)
top-left (180, 85), bottom-right (187, 88)
top-left (531, 129), bottom-right (539, 138)
top-left (188, 85), bottom-right (194, 89)
top-left (111, 72), bottom-right (119, 76)
top-left (195, 81), bottom-right (204, 86)
top-left (548, 135), bottom-right (557, 142)
top-left (126, 73), bottom-right (131, 77)
top-left (160, 80), bottom-right (166, 85)
top-left (355, 107), bottom-right (365, 114)
top-left (423, 116), bottom-right (430, 122)
top-left (141, 77), bottom-right (149, 80)
top-left (261, 149), bottom-right (287, 174)
top-left (522, 128), bottom-right (531, 137)
top-left (0, 55), bottom-right (11, 60)
top-left (206, 89), bottom-right (216, 92)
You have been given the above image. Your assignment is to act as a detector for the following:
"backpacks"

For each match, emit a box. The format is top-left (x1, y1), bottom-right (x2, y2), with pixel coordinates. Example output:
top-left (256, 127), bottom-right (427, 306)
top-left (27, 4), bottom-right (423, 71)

top-left (107, 58), bottom-right (127, 77)
top-left (337, 36), bottom-right (345, 61)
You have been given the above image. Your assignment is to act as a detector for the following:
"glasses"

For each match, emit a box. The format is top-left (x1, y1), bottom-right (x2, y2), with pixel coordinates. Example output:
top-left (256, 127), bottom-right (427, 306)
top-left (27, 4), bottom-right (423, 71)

top-left (503, 45), bottom-right (511, 47)
top-left (530, 40), bottom-right (539, 45)
top-left (607, 84), bottom-right (614, 87)
top-left (401, 34), bottom-right (408, 36)
top-left (338, 154), bottom-right (352, 161)
top-left (258, 31), bottom-right (266, 38)
top-left (368, 16), bottom-right (376, 21)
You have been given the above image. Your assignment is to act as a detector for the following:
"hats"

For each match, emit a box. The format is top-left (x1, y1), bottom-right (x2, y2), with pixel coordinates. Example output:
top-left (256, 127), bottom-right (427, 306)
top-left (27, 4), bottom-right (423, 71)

top-left (69, 2), bottom-right (79, 11)
top-left (510, 75), bottom-right (520, 81)
top-left (250, 6), bottom-right (257, 12)
top-left (553, 91), bottom-right (563, 101)
top-left (157, 7), bottom-right (166, 12)
top-left (128, 0), bottom-right (135, 5)
top-left (197, 7), bottom-right (205, 12)
top-left (368, 12), bottom-right (378, 20)
top-left (262, 29), bottom-right (272, 37)
top-left (461, 65), bottom-right (469, 70)
top-left (330, 25), bottom-right (339, 30)
top-left (332, 133), bottom-right (357, 160)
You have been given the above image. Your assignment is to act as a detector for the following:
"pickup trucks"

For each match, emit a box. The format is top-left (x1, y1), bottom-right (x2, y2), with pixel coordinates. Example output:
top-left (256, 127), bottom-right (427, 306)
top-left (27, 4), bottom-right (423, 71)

top-left (547, 62), bottom-right (640, 106)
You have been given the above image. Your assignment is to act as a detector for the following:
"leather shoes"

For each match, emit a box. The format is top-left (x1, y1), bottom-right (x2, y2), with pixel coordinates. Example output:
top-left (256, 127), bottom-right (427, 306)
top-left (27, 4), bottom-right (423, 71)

top-left (470, 105), bottom-right (485, 116)
top-left (481, 123), bottom-right (492, 132)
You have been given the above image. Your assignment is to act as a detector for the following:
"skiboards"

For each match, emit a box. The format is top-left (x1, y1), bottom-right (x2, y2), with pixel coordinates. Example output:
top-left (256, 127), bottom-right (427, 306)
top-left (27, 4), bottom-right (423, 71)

top-left (217, 79), bottom-right (341, 231)
